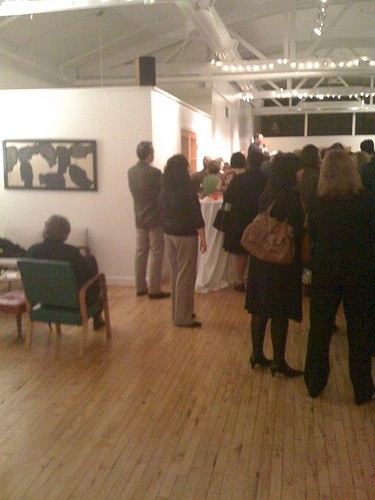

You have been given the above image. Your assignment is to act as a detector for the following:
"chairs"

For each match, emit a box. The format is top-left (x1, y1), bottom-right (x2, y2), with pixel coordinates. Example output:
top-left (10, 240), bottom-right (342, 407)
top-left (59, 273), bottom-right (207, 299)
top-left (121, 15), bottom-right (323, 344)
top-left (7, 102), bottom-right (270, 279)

top-left (17, 258), bottom-right (112, 358)
top-left (0, 257), bottom-right (27, 347)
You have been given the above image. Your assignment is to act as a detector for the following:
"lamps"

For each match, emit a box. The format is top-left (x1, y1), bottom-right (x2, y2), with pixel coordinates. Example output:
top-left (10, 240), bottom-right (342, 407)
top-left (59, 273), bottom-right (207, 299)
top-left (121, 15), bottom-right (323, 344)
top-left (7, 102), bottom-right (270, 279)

top-left (314, 0), bottom-right (328, 36)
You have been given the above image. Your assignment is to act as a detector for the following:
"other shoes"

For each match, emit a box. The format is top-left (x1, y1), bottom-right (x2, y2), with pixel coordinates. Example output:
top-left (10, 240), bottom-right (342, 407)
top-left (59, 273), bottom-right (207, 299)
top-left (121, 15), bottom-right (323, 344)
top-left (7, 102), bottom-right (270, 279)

top-left (93, 320), bottom-right (107, 330)
top-left (136, 289), bottom-right (172, 299)
top-left (174, 312), bottom-right (201, 328)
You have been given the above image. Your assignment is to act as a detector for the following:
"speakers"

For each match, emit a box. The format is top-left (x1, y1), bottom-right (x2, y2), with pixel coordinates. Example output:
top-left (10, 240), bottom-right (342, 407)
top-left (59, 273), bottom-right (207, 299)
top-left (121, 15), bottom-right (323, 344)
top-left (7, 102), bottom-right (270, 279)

top-left (134, 55), bottom-right (156, 87)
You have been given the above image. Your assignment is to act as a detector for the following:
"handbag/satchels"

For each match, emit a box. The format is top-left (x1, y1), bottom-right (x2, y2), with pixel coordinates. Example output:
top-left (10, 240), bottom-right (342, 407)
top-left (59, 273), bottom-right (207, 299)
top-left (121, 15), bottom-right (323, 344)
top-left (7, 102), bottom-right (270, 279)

top-left (240, 196), bottom-right (296, 265)
top-left (212, 202), bottom-right (230, 232)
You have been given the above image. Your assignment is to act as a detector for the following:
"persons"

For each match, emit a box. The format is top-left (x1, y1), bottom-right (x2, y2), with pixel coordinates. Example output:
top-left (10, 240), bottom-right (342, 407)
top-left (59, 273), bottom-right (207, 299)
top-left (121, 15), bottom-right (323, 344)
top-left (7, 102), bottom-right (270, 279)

top-left (242, 152), bottom-right (305, 379)
top-left (128, 140), bottom-right (171, 299)
top-left (26, 215), bottom-right (107, 331)
top-left (248, 132), bottom-right (263, 153)
top-left (301, 148), bottom-right (375, 404)
top-left (194, 138), bottom-right (375, 337)
top-left (157, 155), bottom-right (206, 326)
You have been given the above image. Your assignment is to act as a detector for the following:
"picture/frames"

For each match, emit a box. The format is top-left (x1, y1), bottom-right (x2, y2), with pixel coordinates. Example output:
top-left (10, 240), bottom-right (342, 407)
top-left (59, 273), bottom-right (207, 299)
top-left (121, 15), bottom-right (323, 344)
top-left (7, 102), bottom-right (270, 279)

top-left (3, 139), bottom-right (98, 191)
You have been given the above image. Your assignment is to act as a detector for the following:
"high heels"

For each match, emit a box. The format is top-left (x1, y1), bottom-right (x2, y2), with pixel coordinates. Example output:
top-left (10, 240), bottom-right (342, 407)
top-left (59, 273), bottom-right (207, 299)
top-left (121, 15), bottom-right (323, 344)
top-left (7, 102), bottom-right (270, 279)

top-left (271, 359), bottom-right (304, 378)
top-left (250, 351), bottom-right (272, 370)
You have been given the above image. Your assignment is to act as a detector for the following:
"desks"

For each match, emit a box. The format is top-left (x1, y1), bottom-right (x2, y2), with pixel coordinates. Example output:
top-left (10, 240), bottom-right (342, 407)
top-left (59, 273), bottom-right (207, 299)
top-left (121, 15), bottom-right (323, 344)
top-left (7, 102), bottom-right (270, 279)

top-left (193, 197), bottom-right (232, 293)
